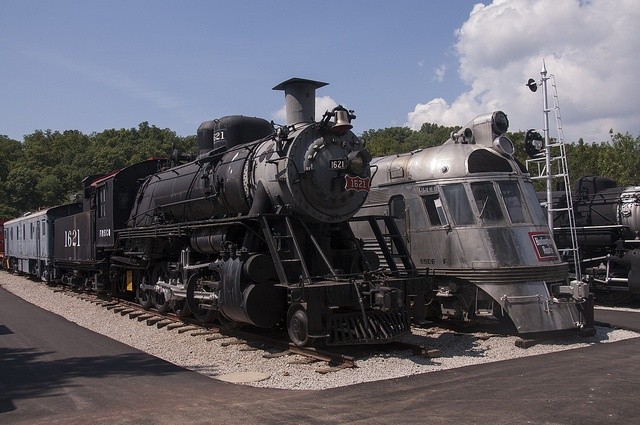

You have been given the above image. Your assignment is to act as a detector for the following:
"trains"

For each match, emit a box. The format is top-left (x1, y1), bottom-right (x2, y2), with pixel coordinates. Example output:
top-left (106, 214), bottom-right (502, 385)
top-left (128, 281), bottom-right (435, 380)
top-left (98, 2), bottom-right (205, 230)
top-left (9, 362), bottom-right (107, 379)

top-left (2, 76), bottom-right (434, 352)
top-left (350, 109), bottom-right (590, 336)
top-left (534, 174), bottom-right (640, 306)
